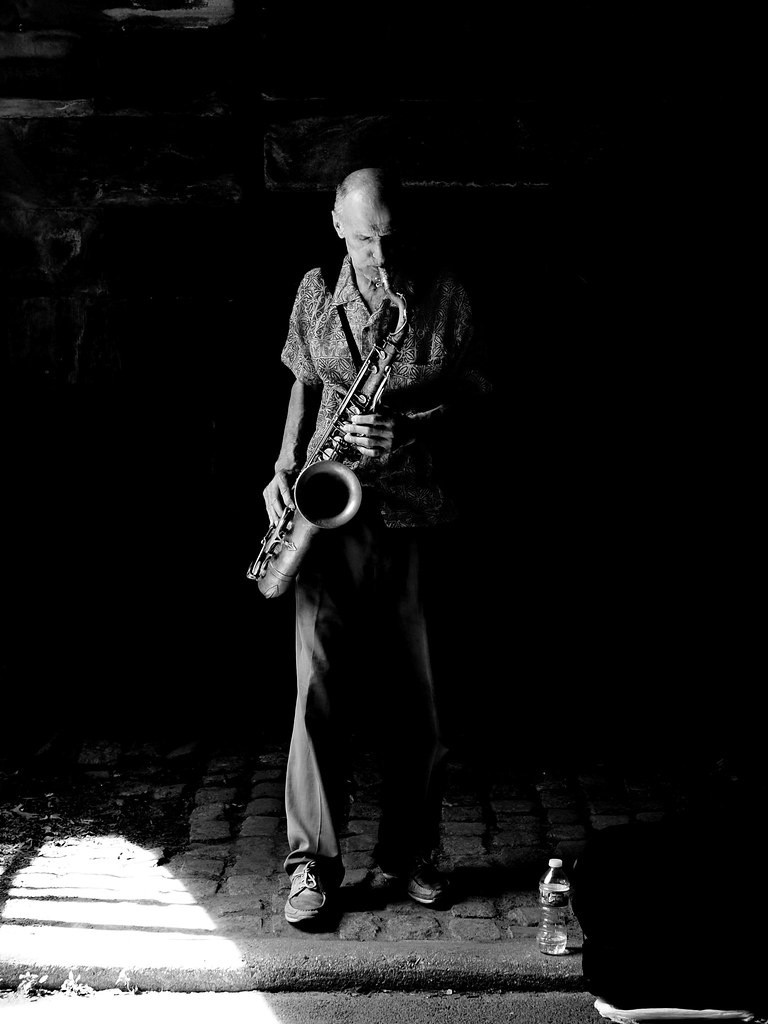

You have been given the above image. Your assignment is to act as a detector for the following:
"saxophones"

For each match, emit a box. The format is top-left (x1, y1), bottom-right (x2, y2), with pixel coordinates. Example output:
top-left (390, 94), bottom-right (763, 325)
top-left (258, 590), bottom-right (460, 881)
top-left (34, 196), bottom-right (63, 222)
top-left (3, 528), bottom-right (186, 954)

top-left (245, 268), bottom-right (408, 600)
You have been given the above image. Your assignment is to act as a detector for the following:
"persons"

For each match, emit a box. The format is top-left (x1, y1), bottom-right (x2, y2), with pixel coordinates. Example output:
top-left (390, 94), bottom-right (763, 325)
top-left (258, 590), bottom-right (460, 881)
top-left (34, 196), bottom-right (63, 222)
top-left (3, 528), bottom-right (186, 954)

top-left (262, 166), bottom-right (502, 926)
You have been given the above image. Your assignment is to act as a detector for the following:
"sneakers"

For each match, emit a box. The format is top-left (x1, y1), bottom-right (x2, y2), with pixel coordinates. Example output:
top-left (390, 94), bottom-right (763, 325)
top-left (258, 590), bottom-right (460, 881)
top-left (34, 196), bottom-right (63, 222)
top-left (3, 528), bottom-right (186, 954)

top-left (381, 862), bottom-right (449, 903)
top-left (284, 862), bottom-right (333, 923)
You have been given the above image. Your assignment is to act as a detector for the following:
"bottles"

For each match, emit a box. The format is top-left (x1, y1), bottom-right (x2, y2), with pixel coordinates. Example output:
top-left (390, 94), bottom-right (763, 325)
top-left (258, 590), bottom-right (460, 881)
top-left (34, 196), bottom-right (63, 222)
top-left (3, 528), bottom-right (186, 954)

top-left (536, 859), bottom-right (571, 955)
